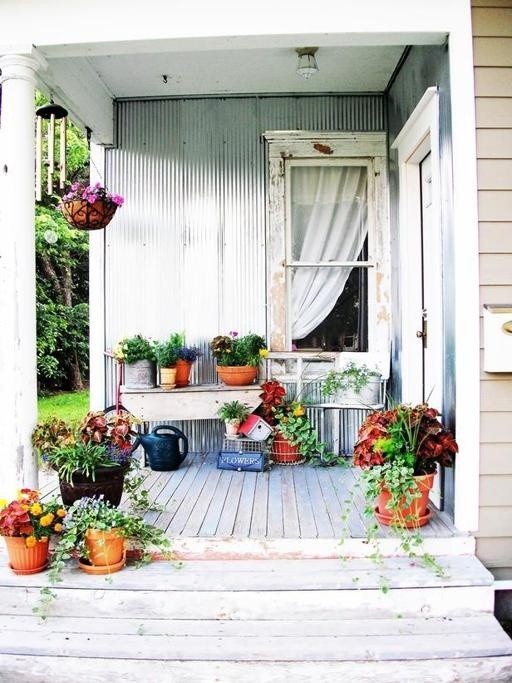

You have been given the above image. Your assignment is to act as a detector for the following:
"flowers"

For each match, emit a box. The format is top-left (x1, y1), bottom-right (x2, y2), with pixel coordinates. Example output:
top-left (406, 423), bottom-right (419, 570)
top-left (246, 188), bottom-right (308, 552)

top-left (29, 412), bottom-right (163, 513)
top-left (209, 328), bottom-right (269, 368)
top-left (61, 183), bottom-right (126, 206)
top-left (31, 493), bottom-right (186, 625)
top-left (174, 345), bottom-right (201, 362)
top-left (259, 377), bottom-right (354, 467)
top-left (0, 482), bottom-right (67, 549)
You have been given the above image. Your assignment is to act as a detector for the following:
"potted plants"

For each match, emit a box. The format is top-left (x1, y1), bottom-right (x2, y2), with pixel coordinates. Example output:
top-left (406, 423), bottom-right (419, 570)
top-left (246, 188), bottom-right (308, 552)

top-left (150, 332), bottom-right (182, 389)
top-left (116, 332), bottom-right (156, 389)
top-left (217, 400), bottom-right (249, 439)
top-left (320, 364), bottom-right (458, 592)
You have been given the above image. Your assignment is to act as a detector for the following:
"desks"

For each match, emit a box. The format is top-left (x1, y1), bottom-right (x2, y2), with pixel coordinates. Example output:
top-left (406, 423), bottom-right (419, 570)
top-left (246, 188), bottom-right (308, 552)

top-left (118, 383), bottom-right (264, 468)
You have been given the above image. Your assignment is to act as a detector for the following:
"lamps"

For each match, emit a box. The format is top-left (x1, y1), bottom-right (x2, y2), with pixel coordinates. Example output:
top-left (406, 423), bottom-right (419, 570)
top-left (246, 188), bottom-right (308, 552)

top-left (295, 46), bottom-right (319, 80)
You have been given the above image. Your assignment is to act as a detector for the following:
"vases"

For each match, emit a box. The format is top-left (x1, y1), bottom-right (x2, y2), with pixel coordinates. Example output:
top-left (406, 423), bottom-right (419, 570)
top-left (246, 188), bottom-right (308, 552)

top-left (3, 531), bottom-right (52, 574)
top-left (167, 358), bottom-right (193, 387)
top-left (70, 527), bottom-right (129, 576)
top-left (259, 425), bottom-right (304, 466)
top-left (215, 364), bottom-right (258, 386)
top-left (51, 466), bottom-right (133, 510)
